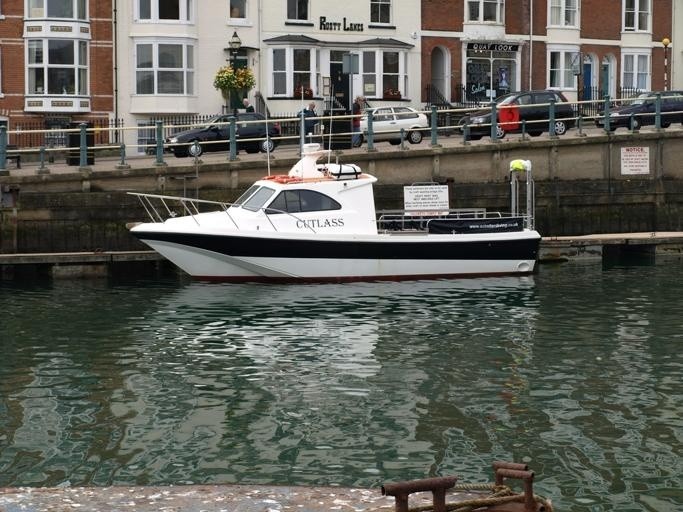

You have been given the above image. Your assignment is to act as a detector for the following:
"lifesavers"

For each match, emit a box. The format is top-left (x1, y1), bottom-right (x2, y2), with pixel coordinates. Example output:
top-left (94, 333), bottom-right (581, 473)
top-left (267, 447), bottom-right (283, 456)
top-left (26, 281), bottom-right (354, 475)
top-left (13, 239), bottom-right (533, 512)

top-left (260, 174), bottom-right (303, 183)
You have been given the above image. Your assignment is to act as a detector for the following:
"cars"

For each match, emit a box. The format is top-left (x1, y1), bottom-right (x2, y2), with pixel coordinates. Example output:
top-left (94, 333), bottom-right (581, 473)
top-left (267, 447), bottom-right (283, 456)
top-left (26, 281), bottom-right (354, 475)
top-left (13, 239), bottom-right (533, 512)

top-left (597, 90), bottom-right (682, 131)
top-left (322, 106), bottom-right (431, 145)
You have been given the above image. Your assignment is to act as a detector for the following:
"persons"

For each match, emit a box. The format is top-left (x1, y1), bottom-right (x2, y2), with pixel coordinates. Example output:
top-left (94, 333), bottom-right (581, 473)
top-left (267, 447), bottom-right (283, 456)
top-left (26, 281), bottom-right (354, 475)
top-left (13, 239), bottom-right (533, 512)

top-left (242, 98), bottom-right (255, 127)
top-left (352, 96), bottom-right (363, 145)
top-left (500, 69), bottom-right (508, 87)
top-left (297, 103), bottom-right (318, 134)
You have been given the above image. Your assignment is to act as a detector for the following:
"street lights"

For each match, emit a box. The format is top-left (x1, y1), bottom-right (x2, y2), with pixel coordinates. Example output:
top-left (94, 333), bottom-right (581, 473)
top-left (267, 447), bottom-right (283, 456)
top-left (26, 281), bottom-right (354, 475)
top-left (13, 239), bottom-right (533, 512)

top-left (225, 31), bottom-right (243, 116)
top-left (662, 38), bottom-right (670, 91)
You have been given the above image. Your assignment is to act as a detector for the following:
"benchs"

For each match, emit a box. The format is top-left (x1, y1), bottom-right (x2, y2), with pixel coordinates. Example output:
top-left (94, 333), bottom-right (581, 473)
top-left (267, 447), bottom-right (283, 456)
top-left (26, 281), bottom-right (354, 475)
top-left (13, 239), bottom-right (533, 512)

top-left (5, 144), bottom-right (20, 168)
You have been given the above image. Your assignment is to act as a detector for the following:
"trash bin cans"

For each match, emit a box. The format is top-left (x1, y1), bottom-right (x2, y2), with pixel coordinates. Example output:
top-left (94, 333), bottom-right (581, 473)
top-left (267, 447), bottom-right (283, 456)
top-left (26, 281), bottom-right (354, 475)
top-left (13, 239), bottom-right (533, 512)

top-left (324, 110), bottom-right (352, 150)
top-left (68, 121), bottom-right (94, 165)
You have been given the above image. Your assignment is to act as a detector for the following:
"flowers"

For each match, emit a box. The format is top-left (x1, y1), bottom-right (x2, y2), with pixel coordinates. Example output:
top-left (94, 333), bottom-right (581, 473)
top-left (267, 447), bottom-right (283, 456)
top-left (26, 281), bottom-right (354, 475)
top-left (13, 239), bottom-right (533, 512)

top-left (211, 66), bottom-right (257, 91)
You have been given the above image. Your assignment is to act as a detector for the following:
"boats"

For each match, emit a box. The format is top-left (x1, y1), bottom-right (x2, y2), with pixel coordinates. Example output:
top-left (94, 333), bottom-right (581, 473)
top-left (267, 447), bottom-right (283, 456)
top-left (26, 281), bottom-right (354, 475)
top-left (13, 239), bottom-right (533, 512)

top-left (126, 140), bottom-right (541, 282)
top-left (144, 280), bottom-right (538, 384)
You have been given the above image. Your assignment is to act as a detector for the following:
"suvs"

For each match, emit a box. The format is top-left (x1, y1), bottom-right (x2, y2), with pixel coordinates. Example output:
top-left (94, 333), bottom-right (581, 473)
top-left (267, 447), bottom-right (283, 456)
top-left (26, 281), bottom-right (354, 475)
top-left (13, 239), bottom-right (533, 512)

top-left (164, 112), bottom-right (281, 157)
top-left (458, 90), bottom-right (574, 137)
